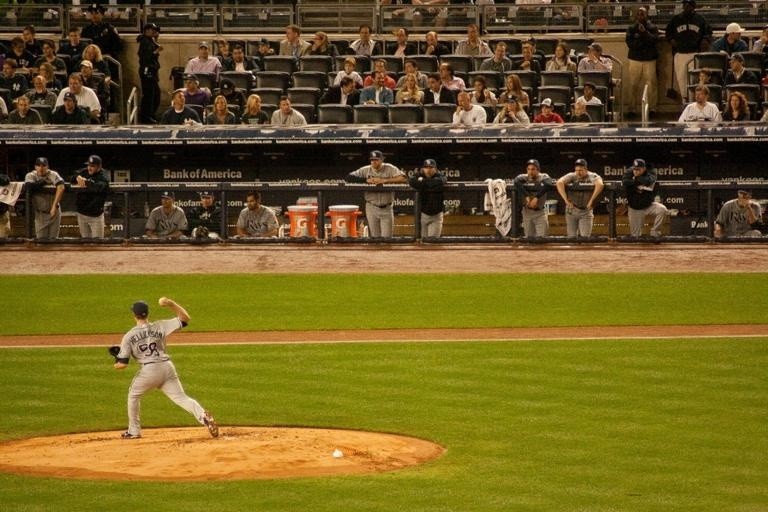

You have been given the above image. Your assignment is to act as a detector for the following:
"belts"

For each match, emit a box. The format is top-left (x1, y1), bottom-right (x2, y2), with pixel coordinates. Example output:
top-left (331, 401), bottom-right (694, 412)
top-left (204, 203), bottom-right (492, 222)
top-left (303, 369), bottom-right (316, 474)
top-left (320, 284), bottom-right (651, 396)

top-left (366, 201), bottom-right (393, 209)
top-left (526, 206), bottom-right (547, 211)
top-left (36, 208), bottom-right (56, 214)
top-left (572, 204), bottom-right (592, 211)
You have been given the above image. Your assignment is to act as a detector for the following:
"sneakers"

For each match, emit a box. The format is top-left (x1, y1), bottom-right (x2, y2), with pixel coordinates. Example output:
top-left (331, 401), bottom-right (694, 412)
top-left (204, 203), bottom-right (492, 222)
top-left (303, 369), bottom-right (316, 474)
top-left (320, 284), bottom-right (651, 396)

top-left (203, 410), bottom-right (219, 437)
top-left (121, 431), bottom-right (141, 439)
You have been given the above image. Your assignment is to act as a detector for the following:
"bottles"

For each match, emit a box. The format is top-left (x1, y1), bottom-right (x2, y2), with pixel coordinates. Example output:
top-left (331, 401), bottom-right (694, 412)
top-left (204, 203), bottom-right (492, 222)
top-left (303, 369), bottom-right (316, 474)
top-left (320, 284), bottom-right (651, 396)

top-left (360, 220), bottom-right (369, 237)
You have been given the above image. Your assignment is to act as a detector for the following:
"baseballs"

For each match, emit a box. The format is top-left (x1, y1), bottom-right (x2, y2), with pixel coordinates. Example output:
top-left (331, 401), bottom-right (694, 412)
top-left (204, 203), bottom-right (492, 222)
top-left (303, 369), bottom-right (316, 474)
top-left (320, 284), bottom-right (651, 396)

top-left (333, 450), bottom-right (343, 457)
top-left (159, 297), bottom-right (166, 305)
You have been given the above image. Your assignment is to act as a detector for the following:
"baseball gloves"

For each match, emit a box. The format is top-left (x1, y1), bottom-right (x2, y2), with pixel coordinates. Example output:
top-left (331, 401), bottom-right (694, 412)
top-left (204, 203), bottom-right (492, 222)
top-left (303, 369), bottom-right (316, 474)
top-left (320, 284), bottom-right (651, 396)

top-left (108, 347), bottom-right (120, 355)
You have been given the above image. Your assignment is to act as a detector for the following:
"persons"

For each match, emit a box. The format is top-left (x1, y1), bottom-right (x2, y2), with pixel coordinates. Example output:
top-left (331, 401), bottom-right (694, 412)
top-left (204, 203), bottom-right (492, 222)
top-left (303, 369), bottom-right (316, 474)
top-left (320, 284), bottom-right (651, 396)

top-left (409, 159), bottom-right (445, 238)
top-left (714, 189), bottom-right (764, 238)
top-left (622, 158), bottom-right (669, 238)
top-left (236, 192), bottom-right (281, 238)
top-left (186, 191), bottom-right (223, 240)
top-left (145, 191), bottom-right (188, 240)
top-left (626, 0), bottom-right (767, 122)
top-left (392, 2), bottom-right (648, 35)
top-left (556, 158), bottom-right (605, 238)
top-left (107, 294), bottom-right (221, 440)
top-left (346, 151), bottom-right (408, 237)
top-left (0, 174), bottom-right (13, 240)
top-left (70, 155), bottom-right (111, 239)
top-left (23, 157), bottom-right (65, 243)
top-left (162, 25), bottom-right (623, 128)
top-left (513, 158), bottom-right (555, 237)
top-left (1, 1), bottom-right (163, 125)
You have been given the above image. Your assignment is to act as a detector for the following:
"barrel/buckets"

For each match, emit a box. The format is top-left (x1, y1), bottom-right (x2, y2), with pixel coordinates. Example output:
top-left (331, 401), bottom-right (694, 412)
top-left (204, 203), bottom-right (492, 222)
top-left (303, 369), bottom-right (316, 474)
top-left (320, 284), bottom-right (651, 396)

top-left (285, 206), bottom-right (318, 237)
top-left (324, 204), bottom-right (362, 237)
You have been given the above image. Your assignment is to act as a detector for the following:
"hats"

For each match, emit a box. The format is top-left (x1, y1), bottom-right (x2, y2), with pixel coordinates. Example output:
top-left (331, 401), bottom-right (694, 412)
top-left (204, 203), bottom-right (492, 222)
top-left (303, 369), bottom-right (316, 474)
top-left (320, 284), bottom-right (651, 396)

top-left (35, 157), bottom-right (48, 166)
top-left (526, 159), bottom-right (540, 169)
top-left (726, 22), bottom-right (745, 34)
top-left (728, 55), bottom-right (744, 61)
top-left (143, 23), bottom-right (158, 32)
top-left (201, 190), bottom-right (213, 197)
top-left (84, 155), bottom-right (102, 165)
top-left (159, 191), bottom-right (175, 199)
top-left (131, 302), bottom-right (148, 312)
top-left (575, 159), bottom-right (587, 166)
top-left (63, 7), bottom-right (106, 101)
top-left (370, 151), bottom-right (382, 159)
top-left (180, 36), bottom-right (272, 94)
top-left (630, 159), bottom-right (645, 168)
top-left (505, 38), bottom-right (602, 108)
top-left (738, 184), bottom-right (752, 193)
top-left (424, 159), bottom-right (436, 167)
